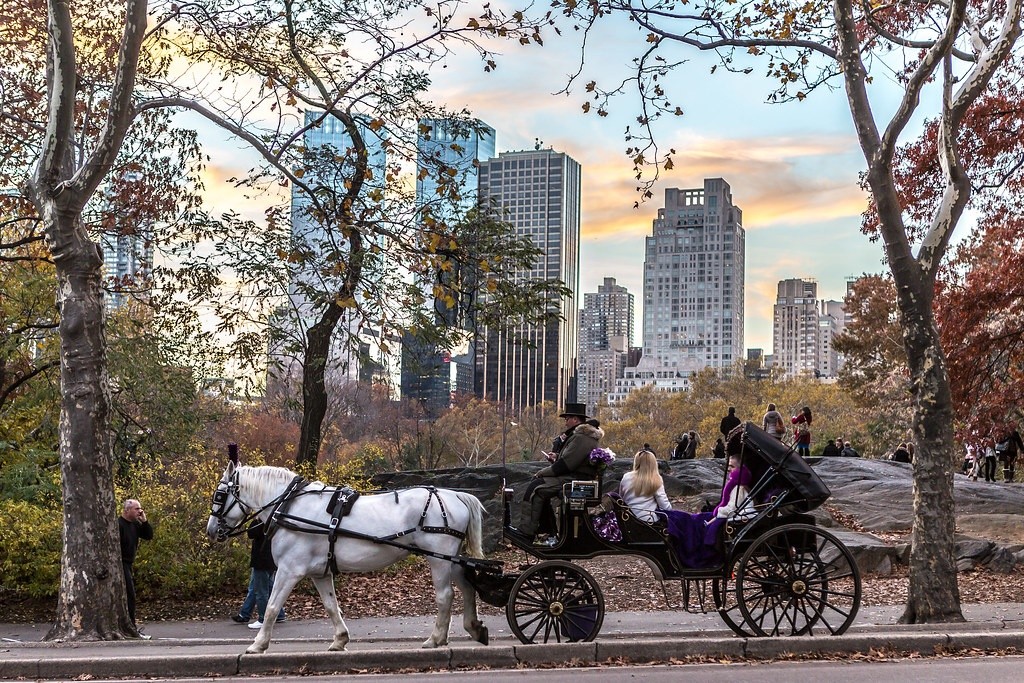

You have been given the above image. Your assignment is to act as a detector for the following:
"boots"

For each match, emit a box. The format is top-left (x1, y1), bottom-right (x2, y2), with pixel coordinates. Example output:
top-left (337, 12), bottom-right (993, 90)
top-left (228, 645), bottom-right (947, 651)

top-left (1009, 470), bottom-right (1014, 480)
top-left (1002, 469), bottom-right (1012, 483)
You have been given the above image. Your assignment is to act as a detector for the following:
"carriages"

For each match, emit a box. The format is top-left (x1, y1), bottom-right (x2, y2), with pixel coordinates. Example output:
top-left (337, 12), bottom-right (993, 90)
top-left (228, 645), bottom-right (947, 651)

top-left (206, 419), bottom-right (863, 651)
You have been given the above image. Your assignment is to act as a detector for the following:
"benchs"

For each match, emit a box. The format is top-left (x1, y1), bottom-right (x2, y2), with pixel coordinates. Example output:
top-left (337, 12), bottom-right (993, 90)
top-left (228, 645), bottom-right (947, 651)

top-left (558, 466), bottom-right (604, 507)
top-left (716, 483), bottom-right (784, 562)
top-left (606, 491), bottom-right (667, 539)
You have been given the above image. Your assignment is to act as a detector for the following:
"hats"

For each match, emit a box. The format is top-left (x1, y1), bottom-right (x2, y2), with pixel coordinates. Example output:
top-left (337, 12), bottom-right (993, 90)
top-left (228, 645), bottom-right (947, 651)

top-left (558, 403), bottom-right (592, 420)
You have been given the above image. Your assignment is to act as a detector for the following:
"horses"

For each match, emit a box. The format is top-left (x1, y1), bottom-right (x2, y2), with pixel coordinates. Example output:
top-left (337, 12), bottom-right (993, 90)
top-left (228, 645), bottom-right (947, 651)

top-left (204, 458), bottom-right (490, 655)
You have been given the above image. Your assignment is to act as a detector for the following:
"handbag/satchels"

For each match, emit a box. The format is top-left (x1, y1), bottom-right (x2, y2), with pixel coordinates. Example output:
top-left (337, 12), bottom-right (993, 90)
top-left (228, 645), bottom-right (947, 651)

top-left (796, 416), bottom-right (809, 434)
top-left (775, 419), bottom-right (786, 433)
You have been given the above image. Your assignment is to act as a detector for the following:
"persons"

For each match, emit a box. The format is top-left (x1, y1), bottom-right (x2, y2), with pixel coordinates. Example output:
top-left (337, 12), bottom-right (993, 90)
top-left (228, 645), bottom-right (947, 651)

top-left (673, 431), bottom-right (696, 459)
top-left (720, 407), bottom-right (741, 441)
top-left (823, 438), bottom-right (860, 457)
top-left (118, 499), bottom-right (153, 640)
top-left (966, 421), bottom-right (1024, 482)
top-left (792, 407), bottom-right (812, 456)
top-left (644, 443), bottom-right (656, 457)
top-left (762, 404), bottom-right (784, 440)
top-left (232, 520), bottom-right (285, 628)
top-left (619, 451), bottom-right (694, 565)
top-left (507, 404), bottom-right (604, 542)
top-left (894, 442), bottom-right (914, 463)
top-left (694, 454), bottom-right (759, 562)
top-left (711, 438), bottom-right (725, 458)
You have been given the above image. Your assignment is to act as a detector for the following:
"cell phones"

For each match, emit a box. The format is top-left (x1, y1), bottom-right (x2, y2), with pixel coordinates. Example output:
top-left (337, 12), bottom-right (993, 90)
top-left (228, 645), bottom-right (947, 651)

top-left (541, 451), bottom-right (549, 458)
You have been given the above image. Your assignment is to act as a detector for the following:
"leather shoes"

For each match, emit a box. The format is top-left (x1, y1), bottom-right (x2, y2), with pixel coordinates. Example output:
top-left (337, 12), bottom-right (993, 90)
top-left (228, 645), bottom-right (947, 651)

top-left (507, 526), bottom-right (535, 544)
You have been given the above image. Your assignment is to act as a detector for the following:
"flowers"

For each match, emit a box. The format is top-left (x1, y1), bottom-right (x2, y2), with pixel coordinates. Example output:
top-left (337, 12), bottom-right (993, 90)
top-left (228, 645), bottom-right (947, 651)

top-left (589, 447), bottom-right (617, 471)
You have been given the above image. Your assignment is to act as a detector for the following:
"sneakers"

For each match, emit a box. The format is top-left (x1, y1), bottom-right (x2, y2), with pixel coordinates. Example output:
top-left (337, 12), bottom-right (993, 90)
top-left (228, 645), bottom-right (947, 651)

top-left (248, 621), bottom-right (263, 629)
top-left (232, 613), bottom-right (250, 623)
